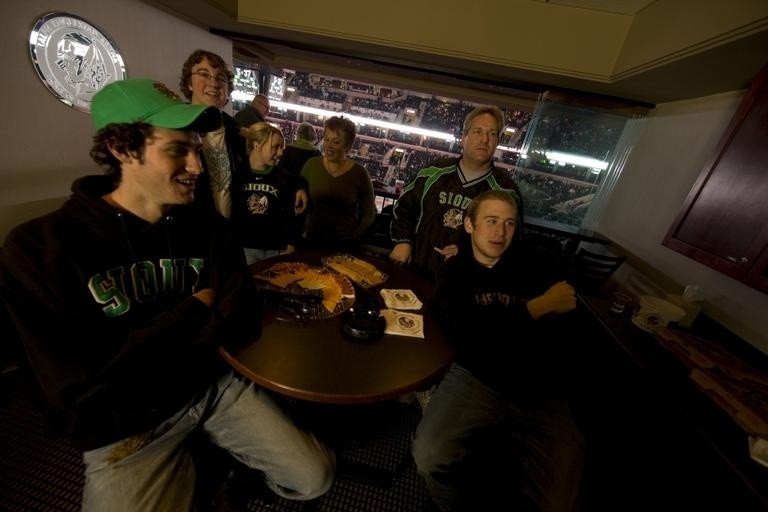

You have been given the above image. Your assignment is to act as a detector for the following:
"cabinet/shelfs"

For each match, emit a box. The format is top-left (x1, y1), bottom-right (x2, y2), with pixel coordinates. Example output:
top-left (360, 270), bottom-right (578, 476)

top-left (660, 63), bottom-right (767, 294)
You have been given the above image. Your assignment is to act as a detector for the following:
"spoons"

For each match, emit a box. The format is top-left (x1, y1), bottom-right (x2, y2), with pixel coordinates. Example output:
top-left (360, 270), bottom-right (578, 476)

top-left (261, 291), bottom-right (320, 318)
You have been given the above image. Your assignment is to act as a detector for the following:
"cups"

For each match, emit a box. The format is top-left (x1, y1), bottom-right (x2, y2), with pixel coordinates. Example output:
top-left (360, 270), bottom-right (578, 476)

top-left (395, 180), bottom-right (404, 194)
top-left (611, 291), bottom-right (632, 314)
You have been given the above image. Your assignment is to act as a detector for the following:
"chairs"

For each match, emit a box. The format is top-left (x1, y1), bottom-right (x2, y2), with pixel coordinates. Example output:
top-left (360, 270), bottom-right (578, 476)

top-left (551, 235), bottom-right (628, 296)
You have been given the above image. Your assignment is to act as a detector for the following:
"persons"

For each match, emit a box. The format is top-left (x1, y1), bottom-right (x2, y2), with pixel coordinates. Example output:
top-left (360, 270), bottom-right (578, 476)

top-left (2, 78), bottom-right (336, 512)
top-left (389, 105), bottom-right (524, 288)
top-left (410, 190), bottom-right (576, 512)
top-left (180, 50), bottom-right (376, 284)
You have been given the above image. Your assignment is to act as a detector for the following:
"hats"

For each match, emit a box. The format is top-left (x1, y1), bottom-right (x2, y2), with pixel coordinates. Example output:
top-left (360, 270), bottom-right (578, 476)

top-left (90, 78), bottom-right (209, 134)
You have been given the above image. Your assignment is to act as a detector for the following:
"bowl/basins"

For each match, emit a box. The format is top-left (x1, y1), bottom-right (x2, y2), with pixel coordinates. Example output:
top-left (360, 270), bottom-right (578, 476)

top-left (344, 305), bottom-right (386, 341)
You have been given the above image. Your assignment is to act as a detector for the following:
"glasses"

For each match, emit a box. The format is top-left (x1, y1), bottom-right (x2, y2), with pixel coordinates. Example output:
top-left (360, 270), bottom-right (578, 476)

top-left (191, 71), bottom-right (230, 85)
top-left (257, 101), bottom-right (270, 111)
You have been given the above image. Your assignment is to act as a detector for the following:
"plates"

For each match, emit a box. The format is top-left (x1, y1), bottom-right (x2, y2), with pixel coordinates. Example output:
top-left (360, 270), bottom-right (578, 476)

top-left (255, 263), bottom-right (356, 321)
